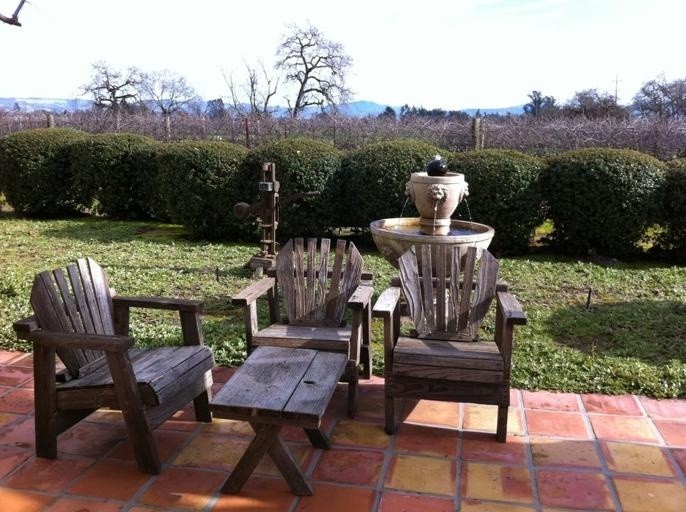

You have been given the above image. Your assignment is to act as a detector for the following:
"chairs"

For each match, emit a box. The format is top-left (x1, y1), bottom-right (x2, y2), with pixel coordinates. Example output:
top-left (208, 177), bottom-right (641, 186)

top-left (230, 238), bottom-right (374, 420)
top-left (13, 255), bottom-right (217, 476)
top-left (371, 242), bottom-right (527, 442)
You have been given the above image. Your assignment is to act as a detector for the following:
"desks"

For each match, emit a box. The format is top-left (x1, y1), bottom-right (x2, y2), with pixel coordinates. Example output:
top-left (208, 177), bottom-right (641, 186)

top-left (207, 344), bottom-right (350, 498)
